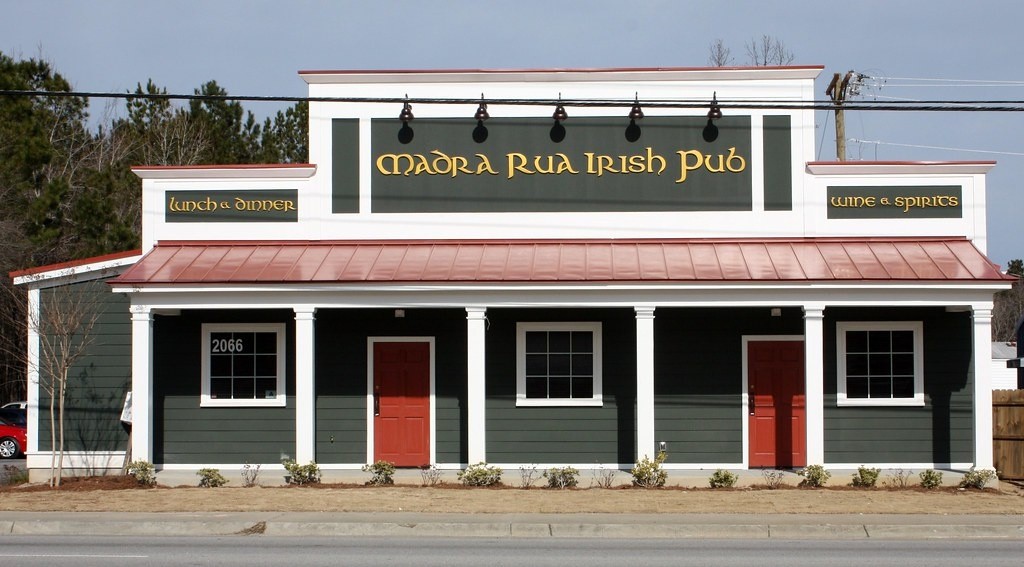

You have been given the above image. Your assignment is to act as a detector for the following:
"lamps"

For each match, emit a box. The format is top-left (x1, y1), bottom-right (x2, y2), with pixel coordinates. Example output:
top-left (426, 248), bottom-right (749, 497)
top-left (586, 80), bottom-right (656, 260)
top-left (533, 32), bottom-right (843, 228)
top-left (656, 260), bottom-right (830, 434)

top-left (400, 94), bottom-right (413, 120)
top-left (553, 92), bottom-right (568, 120)
top-left (475, 93), bottom-right (490, 119)
top-left (629, 92), bottom-right (644, 118)
top-left (708, 91), bottom-right (722, 119)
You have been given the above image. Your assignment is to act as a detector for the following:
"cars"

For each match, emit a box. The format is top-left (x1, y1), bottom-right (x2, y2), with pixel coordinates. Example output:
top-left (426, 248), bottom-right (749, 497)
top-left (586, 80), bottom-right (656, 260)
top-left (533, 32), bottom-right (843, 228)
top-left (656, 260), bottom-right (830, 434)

top-left (0, 401), bottom-right (27, 459)
